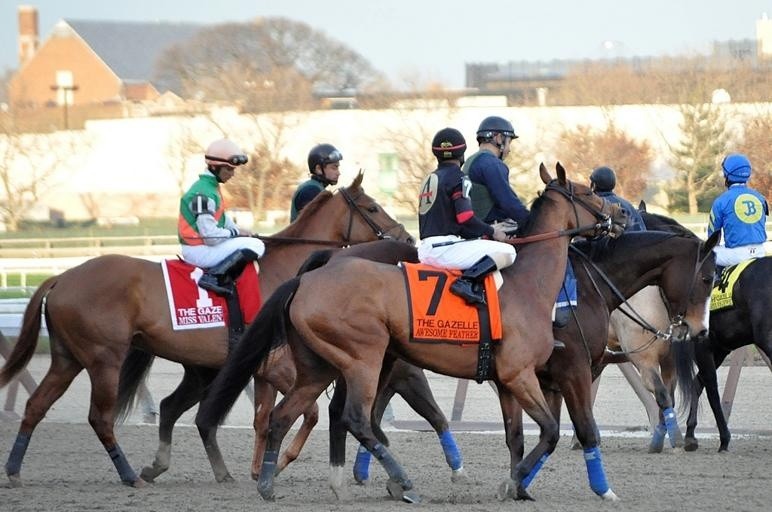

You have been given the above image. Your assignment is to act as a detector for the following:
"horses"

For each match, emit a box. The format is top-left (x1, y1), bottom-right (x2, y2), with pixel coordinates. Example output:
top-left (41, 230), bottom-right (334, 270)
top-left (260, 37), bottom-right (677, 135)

top-left (636, 199), bottom-right (772, 452)
top-left (0, 168), bottom-right (418, 490)
top-left (112, 162), bottom-right (723, 506)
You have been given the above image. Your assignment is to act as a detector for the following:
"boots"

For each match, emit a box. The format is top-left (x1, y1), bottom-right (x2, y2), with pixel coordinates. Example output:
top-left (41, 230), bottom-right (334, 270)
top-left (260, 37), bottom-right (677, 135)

top-left (198, 249), bottom-right (249, 297)
top-left (449, 254), bottom-right (498, 308)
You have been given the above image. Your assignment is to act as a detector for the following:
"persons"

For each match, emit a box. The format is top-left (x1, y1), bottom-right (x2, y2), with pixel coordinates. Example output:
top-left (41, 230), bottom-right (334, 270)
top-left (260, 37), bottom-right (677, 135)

top-left (417, 127), bottom-right (517, 307)
top-left (707, 154), bottom-right (772, 287)
top-left (177, 141), bottom-right (267, 298)
top-left (463, 115), bottom-right (578, 350)
top-left (589, 169), bottom-right (646, 231)
top-left (289, 142), bottom-right (344, 224)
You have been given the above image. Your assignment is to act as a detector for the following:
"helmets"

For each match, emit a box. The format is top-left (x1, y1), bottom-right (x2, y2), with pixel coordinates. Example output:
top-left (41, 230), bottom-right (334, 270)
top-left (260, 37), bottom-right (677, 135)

top-left (205, 138), bottom-right (247, 167)
top-left (590, 166), bottom-right (615, 192)
top-left (722, 153), bottom-right (752, 183)
top-left (477, 116), bottom-right (519, 141)
top-left (308, 144), bottom-right (342, 185)
top-left (432, 127), bottom-right (466, 159)
